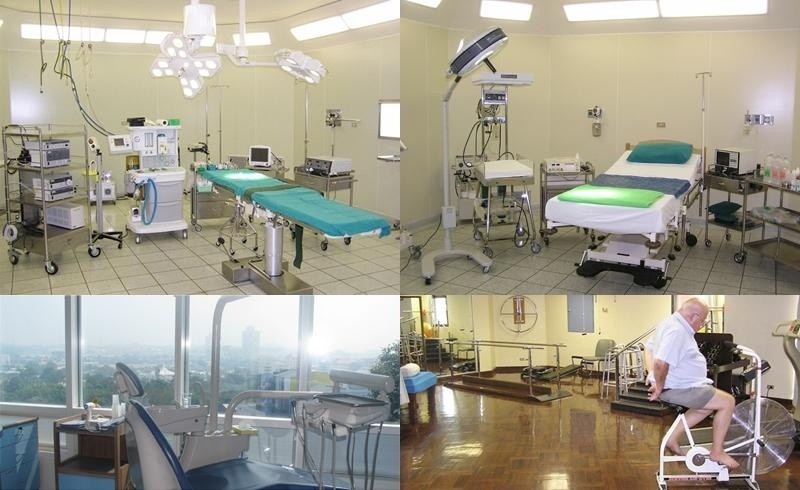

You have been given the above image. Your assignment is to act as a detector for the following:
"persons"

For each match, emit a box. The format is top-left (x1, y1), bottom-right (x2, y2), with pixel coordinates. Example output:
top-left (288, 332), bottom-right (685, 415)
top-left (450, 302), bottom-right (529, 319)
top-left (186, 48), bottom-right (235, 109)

top-left (643, 296), bottom-right (741, 470)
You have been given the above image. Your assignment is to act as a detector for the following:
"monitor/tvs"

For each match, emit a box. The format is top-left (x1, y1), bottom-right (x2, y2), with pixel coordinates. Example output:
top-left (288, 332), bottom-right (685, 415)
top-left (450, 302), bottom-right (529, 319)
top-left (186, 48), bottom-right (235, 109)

top-left (248, 144), bottom-right (271, 166)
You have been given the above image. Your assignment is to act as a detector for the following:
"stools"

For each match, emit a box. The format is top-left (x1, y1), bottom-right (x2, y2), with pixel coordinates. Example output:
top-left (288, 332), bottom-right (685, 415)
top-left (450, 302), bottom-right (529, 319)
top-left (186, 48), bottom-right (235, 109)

top-left (404, 371), bottom-right (438, 410)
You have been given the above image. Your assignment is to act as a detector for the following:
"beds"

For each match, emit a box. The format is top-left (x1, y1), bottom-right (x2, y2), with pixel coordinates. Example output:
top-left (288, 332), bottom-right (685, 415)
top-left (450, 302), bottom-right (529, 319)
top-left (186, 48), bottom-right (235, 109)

top-left (191, 167), bottom-right (391, 295)
top-left (545, 145), bottom-right (706, 289)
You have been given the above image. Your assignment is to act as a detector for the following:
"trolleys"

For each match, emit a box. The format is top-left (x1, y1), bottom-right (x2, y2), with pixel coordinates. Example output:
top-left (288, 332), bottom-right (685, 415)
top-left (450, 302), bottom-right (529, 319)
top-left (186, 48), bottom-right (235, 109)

top-left (473, 167), bottom-right (541, 258)
top-left (294, 167), bottom-right (358, 250)
top-left (3, 124), bottom-right (101, 274)
top-left (703, 163), bottom-right (768, 246)
top-left (539, 162), bottom-right (595, 246)
top-left (734, 170), bottom-right (800, 274)
top-left (190, 169), bottom-right (246, 232)
top-left (249, 169), bottom-right (289, 227)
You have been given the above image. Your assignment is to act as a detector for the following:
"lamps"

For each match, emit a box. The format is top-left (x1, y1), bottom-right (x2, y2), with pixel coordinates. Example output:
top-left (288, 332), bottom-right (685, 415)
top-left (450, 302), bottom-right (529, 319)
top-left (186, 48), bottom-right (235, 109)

top-left (421, 26), bottom-right (509, 285)
top-left (216, 0), bottom-right (328, 83)
top-left (147, 0), bottom-right (222, 100)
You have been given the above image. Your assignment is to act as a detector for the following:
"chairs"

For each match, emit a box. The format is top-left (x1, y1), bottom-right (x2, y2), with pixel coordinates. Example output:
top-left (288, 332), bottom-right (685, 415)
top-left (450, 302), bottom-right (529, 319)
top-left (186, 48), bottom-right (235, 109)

top-left (111, 362), bottom-right (352, 489)
top-left (580, 339), bottom-right (615, 380)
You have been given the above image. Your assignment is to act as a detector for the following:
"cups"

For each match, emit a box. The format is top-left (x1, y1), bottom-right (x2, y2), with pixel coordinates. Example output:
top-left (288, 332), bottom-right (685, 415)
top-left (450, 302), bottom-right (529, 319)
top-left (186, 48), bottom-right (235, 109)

top-left (240, 421), bottom-right (251, 430)
top-left (121, 403), bottom-right (126, 416)
top-left (112, 394), bottom-right (120, 419)
top-left (182, 393), bottom-right (193, 407)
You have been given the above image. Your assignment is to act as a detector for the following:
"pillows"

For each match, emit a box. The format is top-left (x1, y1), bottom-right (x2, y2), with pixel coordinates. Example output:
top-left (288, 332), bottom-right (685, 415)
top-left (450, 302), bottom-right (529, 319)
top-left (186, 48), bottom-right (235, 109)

top-left (626, 139), bottom-right (693, 163)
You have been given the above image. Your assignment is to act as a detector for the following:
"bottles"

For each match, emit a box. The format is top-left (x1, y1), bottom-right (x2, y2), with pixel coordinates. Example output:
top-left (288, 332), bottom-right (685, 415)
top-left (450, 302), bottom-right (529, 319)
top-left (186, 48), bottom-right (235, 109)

top-left (575, 151), bottom-right (581, 173)
top-left (780, 157), bottom-right (792, 188)
top-left (771, 154), bottom-right (782, 185)
top-left (763, 153), bottom-right (774, 184)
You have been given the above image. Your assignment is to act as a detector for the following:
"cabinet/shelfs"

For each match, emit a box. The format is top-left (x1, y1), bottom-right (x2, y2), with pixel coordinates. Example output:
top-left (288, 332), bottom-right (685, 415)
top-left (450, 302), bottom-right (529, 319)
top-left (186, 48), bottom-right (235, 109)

top-left (600, 345), bottom-right (644, 400)
top-left (53, 413), bottom-right (130, 490)
top-left (0, 415), bottom-right (39, 489)
